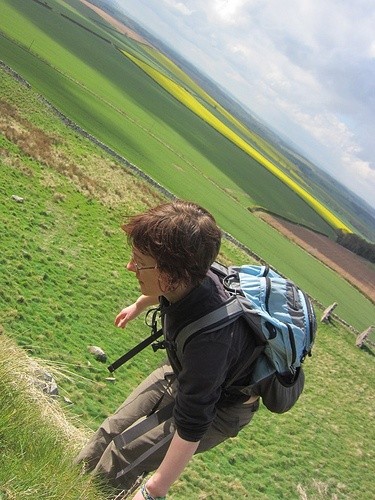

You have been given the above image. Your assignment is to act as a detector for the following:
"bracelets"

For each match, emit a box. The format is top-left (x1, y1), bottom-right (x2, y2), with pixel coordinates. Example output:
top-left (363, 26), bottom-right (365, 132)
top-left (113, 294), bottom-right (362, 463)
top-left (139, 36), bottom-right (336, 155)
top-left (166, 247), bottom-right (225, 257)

top-left (140, 485), bottom-right (168, 500)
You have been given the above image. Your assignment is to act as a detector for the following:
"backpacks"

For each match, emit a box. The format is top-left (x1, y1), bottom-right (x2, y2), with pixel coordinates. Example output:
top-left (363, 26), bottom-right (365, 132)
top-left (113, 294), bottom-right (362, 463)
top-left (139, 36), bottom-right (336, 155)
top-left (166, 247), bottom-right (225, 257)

top-left (171, 261), bottom-right (317, 414)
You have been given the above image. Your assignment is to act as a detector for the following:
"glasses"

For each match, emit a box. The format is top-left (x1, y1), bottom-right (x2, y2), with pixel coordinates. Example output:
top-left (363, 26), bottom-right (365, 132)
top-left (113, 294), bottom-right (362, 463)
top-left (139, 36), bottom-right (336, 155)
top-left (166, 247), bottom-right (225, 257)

top-left (131, 256), bottom-right (156, 272)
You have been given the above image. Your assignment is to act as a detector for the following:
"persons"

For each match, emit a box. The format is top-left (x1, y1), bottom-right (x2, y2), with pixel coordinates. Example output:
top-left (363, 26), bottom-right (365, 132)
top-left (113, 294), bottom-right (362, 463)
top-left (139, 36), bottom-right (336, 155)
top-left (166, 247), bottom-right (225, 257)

top-left (62, 201), bottom-right (262, 500)
